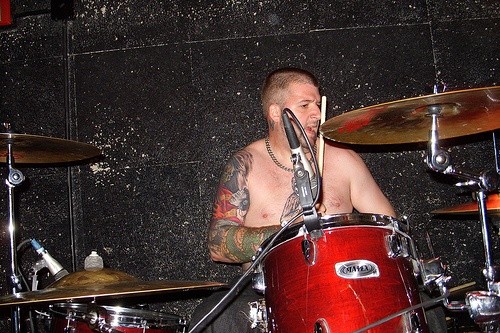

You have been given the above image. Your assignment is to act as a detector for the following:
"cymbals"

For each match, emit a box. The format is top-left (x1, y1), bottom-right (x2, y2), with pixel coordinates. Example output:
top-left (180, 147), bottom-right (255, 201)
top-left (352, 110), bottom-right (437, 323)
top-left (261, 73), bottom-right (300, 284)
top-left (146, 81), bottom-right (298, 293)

top-left (319, 85), bottom-right (500, 146)
top-left (430, 194), bottom-right (500, 215)
top-left (0, 133), bottom-right (103, 163)
top-left (0, 267), bottom-right (230, 308)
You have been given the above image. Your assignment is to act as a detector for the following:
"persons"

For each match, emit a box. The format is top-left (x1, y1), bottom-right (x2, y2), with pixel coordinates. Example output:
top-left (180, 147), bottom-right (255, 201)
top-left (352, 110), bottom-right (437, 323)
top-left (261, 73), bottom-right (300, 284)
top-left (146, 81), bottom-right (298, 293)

top-left (187, 67), bottom-right (448, 333)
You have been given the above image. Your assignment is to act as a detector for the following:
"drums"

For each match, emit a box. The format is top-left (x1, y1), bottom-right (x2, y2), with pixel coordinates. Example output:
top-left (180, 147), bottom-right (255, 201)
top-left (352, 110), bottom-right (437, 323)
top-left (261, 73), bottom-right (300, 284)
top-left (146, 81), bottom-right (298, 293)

top-left (49, 303), bottom-right (189, 333)
top-left (256, 213), bottom-right (429, 333)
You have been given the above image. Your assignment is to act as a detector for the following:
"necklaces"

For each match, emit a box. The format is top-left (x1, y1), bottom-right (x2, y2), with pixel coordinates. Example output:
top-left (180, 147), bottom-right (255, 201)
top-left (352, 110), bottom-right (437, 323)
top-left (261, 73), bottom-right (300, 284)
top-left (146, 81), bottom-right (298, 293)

top-left (264, 135), bottom-right (318, 172)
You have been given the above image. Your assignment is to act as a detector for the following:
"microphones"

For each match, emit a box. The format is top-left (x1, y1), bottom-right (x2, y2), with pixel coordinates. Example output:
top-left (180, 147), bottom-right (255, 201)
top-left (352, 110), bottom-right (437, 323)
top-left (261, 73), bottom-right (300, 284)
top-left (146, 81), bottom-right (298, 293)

top-left (282, 111), bottom-right (318, 199)
top-left (31, 240), bottom-right (69, 281)
